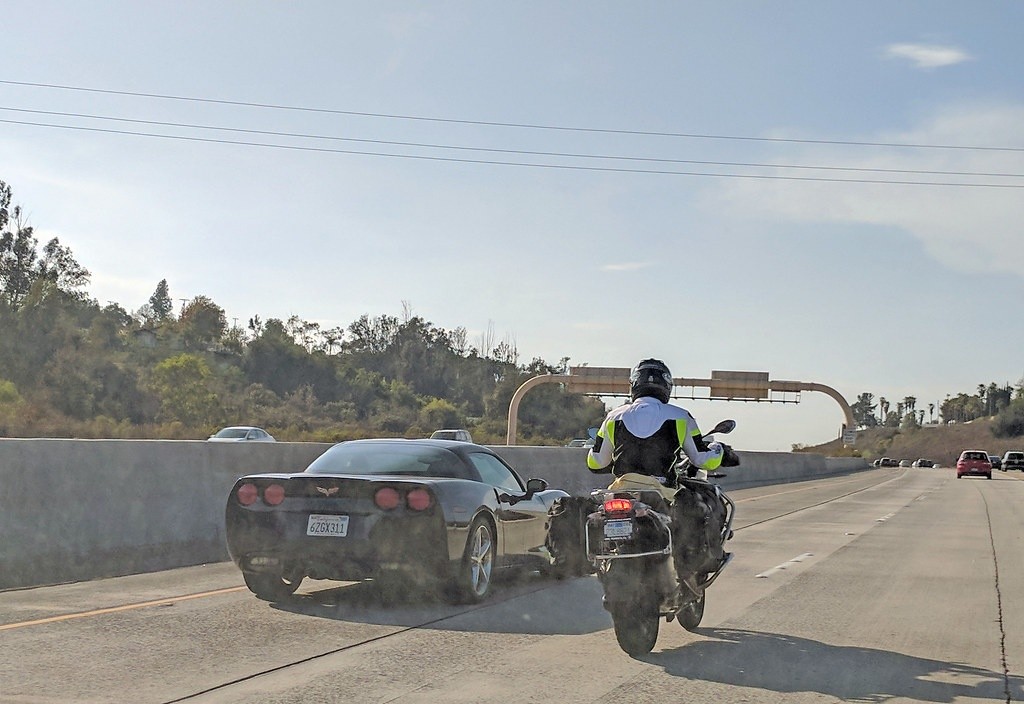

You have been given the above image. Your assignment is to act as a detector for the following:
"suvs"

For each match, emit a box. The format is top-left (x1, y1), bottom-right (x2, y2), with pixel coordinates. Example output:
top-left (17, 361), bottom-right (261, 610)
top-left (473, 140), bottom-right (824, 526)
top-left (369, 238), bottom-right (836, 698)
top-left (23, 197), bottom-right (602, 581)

top-left (998, 450), bottom-right (1024, 472)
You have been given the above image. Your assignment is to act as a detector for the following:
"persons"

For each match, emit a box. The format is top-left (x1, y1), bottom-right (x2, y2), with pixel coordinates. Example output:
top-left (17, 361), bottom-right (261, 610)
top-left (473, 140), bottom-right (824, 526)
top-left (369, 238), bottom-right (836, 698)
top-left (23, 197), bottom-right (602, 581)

top-left (586, 358), bottom-right (729, 575)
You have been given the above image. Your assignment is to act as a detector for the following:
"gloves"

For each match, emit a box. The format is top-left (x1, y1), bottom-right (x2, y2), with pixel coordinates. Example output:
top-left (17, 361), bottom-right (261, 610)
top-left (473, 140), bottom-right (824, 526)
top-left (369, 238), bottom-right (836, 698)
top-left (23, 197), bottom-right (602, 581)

top-left (719, 442), bottom-right (740, 467)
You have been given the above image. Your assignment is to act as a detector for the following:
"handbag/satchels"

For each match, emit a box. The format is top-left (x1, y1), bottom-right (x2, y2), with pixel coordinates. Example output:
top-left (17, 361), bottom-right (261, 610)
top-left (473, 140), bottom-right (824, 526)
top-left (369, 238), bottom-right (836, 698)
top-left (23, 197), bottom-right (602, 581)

top-left (676, 487), bottom-right (724, 573)
top-left (545, 496), bottom-right (601, 576)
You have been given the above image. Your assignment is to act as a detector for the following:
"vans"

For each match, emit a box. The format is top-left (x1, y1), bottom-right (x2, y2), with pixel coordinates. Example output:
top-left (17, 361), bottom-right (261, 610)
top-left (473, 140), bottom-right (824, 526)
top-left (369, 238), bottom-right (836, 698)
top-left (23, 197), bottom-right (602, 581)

top-left (428, 428), bottom-right (473, 443)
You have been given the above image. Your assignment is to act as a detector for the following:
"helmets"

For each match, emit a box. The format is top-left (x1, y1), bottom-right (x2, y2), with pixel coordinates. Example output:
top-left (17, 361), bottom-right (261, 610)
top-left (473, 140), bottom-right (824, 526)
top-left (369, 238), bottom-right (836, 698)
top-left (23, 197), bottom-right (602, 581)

top-left (629, 358), bottom-right (673, 404)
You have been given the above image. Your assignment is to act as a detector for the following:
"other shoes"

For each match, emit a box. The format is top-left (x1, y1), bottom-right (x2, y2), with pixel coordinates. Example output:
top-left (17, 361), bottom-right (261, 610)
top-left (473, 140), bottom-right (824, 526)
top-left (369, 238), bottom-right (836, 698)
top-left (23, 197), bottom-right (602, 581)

top-left (680, 570), bottom-right (703, 597)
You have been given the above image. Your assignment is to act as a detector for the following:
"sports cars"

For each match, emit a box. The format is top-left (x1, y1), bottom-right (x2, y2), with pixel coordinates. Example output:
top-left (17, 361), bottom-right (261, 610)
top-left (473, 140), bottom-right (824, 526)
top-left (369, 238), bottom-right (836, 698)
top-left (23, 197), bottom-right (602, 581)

top-left (221, 435), bottom-right (572, 606)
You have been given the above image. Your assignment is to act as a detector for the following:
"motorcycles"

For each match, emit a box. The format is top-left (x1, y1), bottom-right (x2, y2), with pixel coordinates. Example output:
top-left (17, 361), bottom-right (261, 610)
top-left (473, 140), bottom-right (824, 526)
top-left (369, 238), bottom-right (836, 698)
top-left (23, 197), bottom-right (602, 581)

top-left (582, 417), bottom-right (741, 656)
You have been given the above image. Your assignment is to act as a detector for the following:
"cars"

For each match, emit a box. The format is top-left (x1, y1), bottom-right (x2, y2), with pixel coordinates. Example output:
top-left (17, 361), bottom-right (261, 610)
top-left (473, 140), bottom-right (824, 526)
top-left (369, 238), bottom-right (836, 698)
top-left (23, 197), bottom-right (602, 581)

top-left (564, 437), bottom-right (588, 447)
top-left (205, 426), bottom-right (277, 443)
top-left (873, 456), bottom-right (943, 469)
top-left (988, 455), bottom-right (1001, 469)
top-left (582, 437), bottom-right (596, 447)
top-left (955, 449), bottom-right (993, 480)
